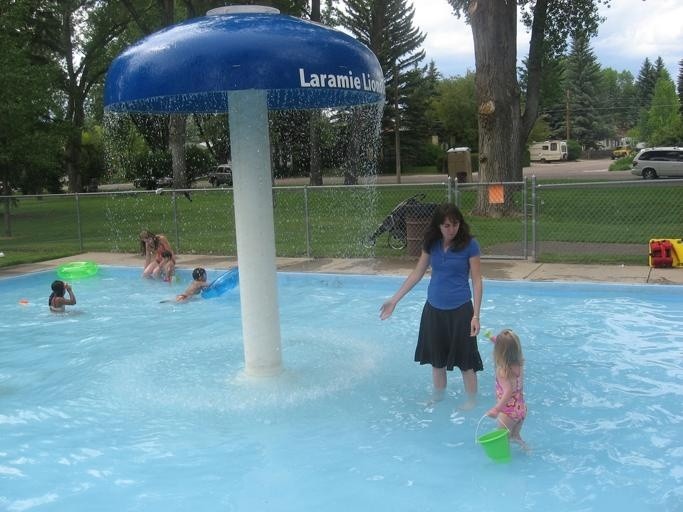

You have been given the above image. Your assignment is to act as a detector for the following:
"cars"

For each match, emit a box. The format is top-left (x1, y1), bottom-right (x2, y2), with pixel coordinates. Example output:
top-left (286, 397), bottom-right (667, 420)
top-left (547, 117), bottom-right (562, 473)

top-left (132, 175), bottom-right (173, 190)
top-left (609, 144), bottom-right (633, 161)
top-left (207, 164), bottom-right (232, 188)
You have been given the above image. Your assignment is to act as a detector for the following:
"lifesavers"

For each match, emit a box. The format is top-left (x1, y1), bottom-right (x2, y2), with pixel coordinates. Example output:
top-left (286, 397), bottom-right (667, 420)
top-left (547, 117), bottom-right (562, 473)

top-left (201, 267), bottom-right (239, 300)
top-left (57, 260), bottom-right (98, 279)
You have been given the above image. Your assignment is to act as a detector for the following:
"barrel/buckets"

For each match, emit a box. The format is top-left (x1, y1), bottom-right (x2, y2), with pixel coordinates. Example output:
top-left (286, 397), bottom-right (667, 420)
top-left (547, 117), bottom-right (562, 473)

top-left (475, 414), bottom-right (511, 462)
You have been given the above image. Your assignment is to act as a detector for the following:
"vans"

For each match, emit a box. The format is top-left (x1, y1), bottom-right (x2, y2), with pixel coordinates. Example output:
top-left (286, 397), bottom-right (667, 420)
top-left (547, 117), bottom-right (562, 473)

top-left (631, 146), bottom-right (682, 180)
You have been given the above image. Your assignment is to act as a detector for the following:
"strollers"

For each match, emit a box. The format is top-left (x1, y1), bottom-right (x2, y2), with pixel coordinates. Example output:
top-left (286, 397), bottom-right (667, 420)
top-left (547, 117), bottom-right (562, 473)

top-left (362, 192), bottom-right (437, 250)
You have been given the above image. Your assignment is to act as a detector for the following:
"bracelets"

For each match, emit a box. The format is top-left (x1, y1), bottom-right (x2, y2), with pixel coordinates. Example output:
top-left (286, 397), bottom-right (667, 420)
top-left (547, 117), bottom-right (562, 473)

top-left (472, 316), bottom-right (479, 320)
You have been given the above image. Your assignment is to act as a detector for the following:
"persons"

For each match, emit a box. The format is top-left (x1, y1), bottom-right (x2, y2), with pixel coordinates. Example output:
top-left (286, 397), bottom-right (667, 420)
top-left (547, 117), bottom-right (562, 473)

top-left (487, 328), bottom-right (528, 441)
top-left (139, 230), bottom-right (175, 280)
top-left (48, 280), bottom-right (76, 312)
top-left (174, 267), bottom-right (211, 301)
top-left (379, 202), bottom-right (484, 409)
top-left (159, 250), bottom-right (174, 283)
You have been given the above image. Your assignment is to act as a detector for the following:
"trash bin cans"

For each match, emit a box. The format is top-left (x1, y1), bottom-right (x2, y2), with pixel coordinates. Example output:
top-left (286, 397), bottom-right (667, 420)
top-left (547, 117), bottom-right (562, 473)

top-left (446, 147), bottom-right (472, 183)
